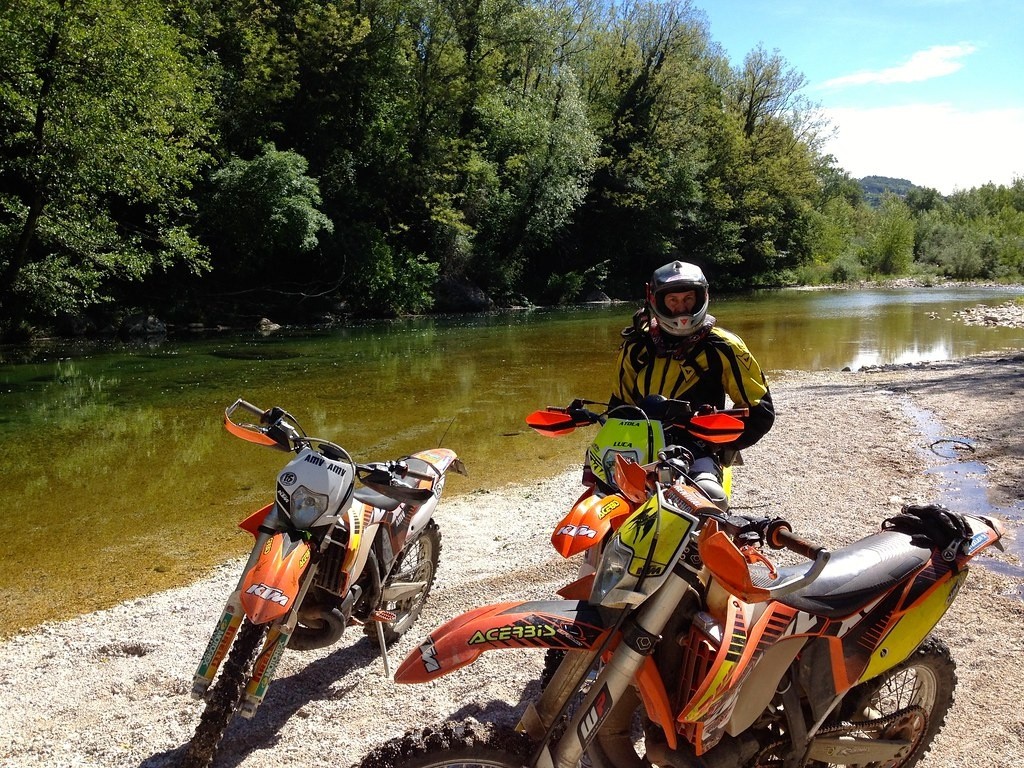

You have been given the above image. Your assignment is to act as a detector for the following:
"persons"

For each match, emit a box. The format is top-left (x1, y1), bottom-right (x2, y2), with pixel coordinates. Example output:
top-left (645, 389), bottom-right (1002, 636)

top-left (612, 261), bottom-right (775, 511)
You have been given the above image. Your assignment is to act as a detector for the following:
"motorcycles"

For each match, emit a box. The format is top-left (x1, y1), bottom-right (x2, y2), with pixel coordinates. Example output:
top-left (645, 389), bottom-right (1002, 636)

top-left (189, 398), bottom-right (469, 768)
top-left (525, 389), bottom-right (750, 688)
top-left (349, 447), bottom-right (1009, 767)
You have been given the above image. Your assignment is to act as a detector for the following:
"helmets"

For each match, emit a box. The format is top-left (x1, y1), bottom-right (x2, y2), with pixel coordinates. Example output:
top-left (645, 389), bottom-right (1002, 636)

top-left (649, 260), bottom-right (709, 337)
